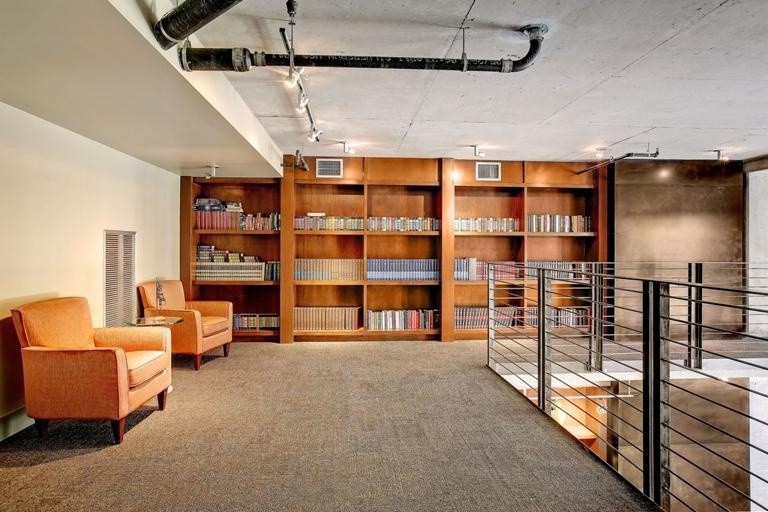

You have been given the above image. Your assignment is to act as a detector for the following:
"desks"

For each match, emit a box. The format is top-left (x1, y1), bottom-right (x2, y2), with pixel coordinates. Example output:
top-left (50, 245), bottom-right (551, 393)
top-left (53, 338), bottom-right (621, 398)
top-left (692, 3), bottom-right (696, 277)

top-left (124, 315), bottom-right (184, 330)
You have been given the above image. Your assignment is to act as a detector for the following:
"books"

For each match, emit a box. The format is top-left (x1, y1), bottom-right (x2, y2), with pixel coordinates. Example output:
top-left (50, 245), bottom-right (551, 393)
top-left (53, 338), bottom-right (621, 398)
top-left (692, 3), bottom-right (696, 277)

top-left (453, 214), bottom-right (594, 234)
top-left (454, 257), bottom-right (599, 280)
top-left (293, 305), bottom-right (441, 332)
top-left (293, 256), bottom-right (440, 282)
top-left (232, 313), bottom-right (280, 331)
top-left (194, 198), bottom-right (281, 230)
top-left (294, 212), bottom-right (440, 231)
top-left (453, 306), bottom-right (594, 330)
top-left (194, 245), bottom-right (280, 282)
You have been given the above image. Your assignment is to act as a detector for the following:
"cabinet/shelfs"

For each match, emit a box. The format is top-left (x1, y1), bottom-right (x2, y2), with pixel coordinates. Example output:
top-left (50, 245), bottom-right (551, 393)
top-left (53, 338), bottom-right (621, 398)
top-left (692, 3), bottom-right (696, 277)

top-left (440, 156), bottom-right (607, 343)
top-left (281, 152), bottom-right (440, 344)
top-left (178, 173), bottom-right (280, 340)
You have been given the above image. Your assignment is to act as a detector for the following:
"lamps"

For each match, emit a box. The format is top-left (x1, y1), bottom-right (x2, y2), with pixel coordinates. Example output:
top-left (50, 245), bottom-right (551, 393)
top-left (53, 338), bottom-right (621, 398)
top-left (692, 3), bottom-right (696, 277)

top-left (280, 23), bottom-right (323, 144)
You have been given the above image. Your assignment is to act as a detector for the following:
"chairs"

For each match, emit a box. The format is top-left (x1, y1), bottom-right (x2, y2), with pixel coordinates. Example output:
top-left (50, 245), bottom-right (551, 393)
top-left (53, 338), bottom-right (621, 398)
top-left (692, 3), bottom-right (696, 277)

top-left (137, 279), bottom-right (233, 371)
top-left (8, 295), bottom-right (176, 444)
